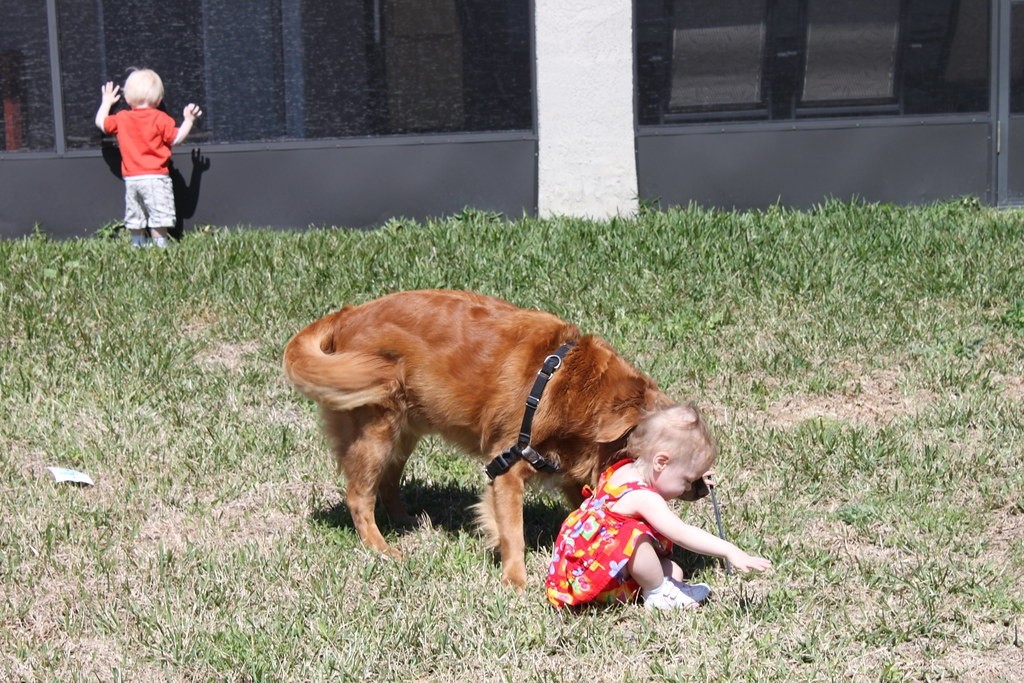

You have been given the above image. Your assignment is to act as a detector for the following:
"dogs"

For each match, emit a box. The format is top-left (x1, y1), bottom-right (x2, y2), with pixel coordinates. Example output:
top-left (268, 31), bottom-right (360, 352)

top-left (281, 288), bottom-right (710, 588)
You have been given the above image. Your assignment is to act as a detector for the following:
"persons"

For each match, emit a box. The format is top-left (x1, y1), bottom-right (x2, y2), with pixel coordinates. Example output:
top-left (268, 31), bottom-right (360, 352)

top-left (545, 398), bottom-right (772, 610)
top-left (95, 70), bottom-right (202, 250)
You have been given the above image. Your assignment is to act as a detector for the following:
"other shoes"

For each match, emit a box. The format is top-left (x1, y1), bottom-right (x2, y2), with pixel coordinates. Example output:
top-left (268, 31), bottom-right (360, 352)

top-left (643, 575), bottom-right (712, 613)
